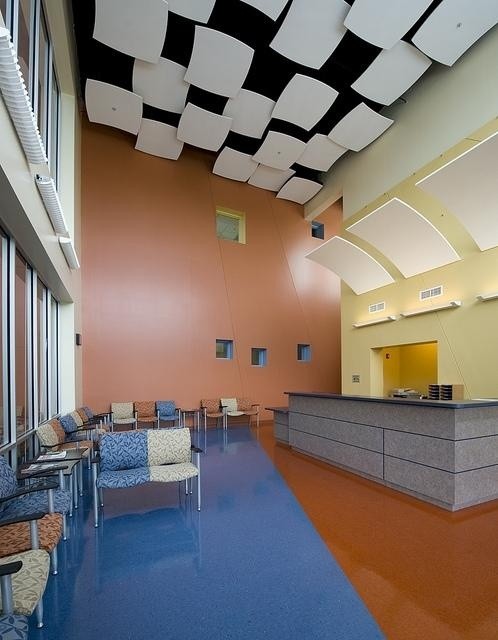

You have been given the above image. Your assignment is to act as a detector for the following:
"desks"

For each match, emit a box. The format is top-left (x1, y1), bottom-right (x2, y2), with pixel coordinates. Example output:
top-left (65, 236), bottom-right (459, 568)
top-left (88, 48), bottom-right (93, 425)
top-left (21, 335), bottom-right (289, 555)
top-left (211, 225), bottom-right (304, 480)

top-left (17, 449), bottom-right (86, 540)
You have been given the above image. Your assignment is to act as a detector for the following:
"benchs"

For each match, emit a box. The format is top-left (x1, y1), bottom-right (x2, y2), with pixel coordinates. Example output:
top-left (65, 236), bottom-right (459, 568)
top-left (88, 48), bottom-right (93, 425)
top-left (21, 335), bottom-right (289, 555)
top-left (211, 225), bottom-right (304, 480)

top-left (92, 428), bottom-right (201, 529)
top-left (1, 452), bottom-right (72, 640)
top-left (34, 406), bottom-right (115, 466)
top-left (110, 397), bottom-right (259, 432)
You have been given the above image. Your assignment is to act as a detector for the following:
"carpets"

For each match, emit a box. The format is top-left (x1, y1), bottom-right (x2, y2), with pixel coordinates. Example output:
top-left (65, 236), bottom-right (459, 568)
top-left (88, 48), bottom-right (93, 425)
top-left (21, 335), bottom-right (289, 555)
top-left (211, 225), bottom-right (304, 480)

top-left (14, 426), bottom-right (386, 639)
